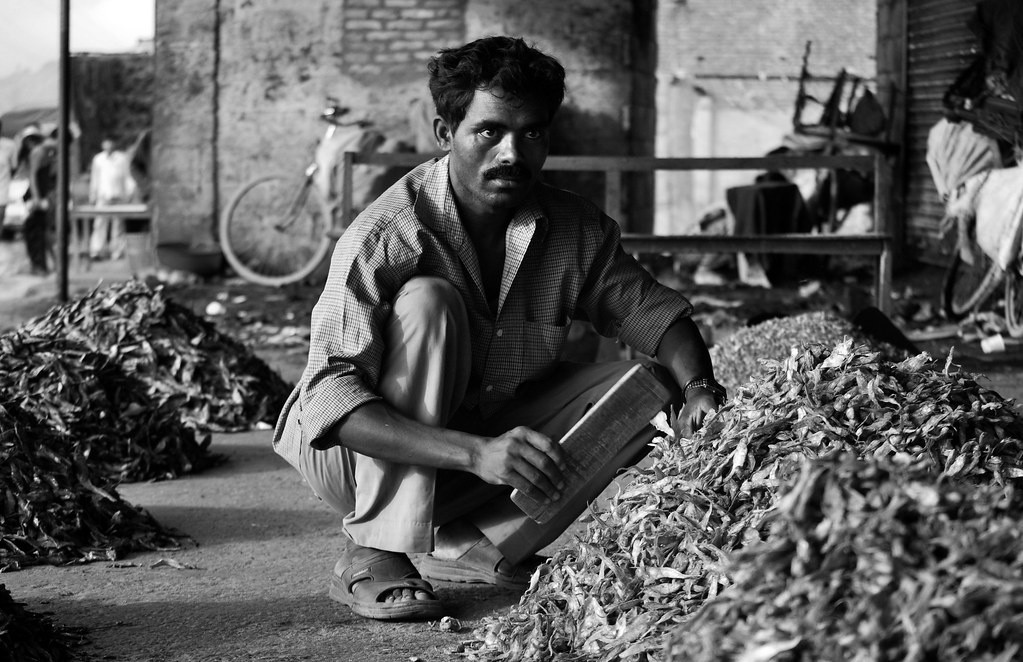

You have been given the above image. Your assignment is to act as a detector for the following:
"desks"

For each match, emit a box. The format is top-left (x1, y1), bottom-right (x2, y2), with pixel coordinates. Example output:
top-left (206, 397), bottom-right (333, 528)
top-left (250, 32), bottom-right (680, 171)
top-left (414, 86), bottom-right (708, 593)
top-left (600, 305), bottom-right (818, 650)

top-left (68, 204), bottom-right (149, 272)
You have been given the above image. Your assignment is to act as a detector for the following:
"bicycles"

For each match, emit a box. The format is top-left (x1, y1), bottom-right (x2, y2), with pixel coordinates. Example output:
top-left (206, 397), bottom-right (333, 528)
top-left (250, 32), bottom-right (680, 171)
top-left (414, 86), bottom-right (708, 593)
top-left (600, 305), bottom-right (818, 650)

top-left (218, 97), bottom-right (404, 289)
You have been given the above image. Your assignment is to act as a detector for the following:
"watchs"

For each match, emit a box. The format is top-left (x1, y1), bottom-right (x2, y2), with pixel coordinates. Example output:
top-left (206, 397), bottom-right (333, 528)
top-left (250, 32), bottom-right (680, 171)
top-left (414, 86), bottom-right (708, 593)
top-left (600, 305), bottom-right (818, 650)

top-left (682, 377), bottom-right (727, 406)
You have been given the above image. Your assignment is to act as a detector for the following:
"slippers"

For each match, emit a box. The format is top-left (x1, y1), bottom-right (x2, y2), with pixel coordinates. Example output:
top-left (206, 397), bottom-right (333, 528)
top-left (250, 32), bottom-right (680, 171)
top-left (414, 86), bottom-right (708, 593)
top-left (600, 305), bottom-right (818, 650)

top-left (329, 547), bottom-right (442, 619)
top-left (420, 535), bottom-right (552, 591)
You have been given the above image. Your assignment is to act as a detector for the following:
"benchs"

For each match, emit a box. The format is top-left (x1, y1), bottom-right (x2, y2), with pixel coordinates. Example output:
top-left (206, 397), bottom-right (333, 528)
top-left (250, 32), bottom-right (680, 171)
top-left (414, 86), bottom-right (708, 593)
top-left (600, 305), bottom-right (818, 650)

top-left (341, 150), bottom-right (896, 319)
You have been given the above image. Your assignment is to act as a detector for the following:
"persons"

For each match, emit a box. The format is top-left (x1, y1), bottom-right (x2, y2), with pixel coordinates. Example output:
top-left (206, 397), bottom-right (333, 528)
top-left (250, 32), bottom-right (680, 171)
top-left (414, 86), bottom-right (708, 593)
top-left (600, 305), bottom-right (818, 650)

top-left (21, 126), bottom-right (74, 274)
top-left (272, 36), bottom-right (726, 620)
top-left (674, 171), bottom-right (787, 288)
top-left (0, 120), bottom-right (44, 242)
top-left (88, 134), bottom-right (134, 261)
